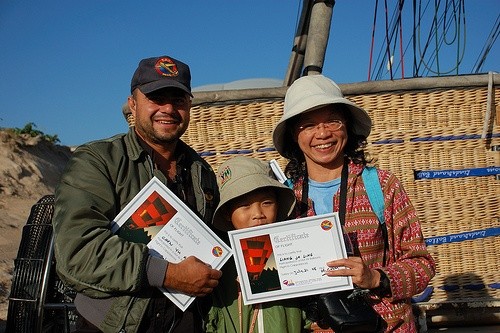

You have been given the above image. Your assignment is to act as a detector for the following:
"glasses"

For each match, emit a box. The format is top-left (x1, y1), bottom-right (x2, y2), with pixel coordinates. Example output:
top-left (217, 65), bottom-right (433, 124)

top-left (296, 120), bottom-right (348, 134)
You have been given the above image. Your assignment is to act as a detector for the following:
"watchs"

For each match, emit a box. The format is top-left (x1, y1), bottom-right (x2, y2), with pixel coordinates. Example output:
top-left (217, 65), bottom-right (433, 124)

top-left (375, 269), bottom-right (389, 290)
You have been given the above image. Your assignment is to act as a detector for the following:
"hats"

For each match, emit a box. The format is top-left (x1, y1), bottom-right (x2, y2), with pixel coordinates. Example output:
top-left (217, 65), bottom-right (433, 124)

top-left (273, 75), bottom-right (371, 160)
top-left (212, 156), bottom-right (296, 231)
top-left (131, 56), bottom-right (194, 98)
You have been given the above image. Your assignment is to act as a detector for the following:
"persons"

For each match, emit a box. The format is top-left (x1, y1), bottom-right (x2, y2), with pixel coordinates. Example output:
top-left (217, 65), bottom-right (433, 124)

top-left (272, 74), bottom-right (436, 333)
top-left (53, 55), bottom-right (222, 333)
top-left (204, 156), bottom-right (313, 333)
